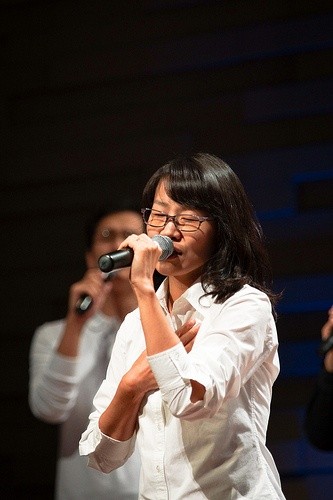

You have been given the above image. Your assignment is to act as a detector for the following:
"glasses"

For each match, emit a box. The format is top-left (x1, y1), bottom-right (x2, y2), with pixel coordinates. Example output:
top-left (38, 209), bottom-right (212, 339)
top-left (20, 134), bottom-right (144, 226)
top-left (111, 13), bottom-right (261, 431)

top-left (141, 206), bottom-right (216, 231)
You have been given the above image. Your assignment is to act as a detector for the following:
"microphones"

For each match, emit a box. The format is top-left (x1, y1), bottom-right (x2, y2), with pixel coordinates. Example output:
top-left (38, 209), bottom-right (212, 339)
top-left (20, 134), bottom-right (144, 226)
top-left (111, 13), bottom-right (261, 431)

top-left (75, 270), bottom-right (113, 314)
top-left (98, 234), bottom-right (174, 273)
top-left (318, 334), bottom-right (333, 356)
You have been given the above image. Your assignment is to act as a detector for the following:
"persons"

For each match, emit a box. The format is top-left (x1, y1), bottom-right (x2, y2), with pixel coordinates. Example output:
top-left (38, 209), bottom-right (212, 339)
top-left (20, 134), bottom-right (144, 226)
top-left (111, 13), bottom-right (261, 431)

top-left (30, 194), bottom-right (160, 500)
top-left (76, 150), bottom-right (288, 500)
top-left (321, 307), bottom-right (333, 373)
